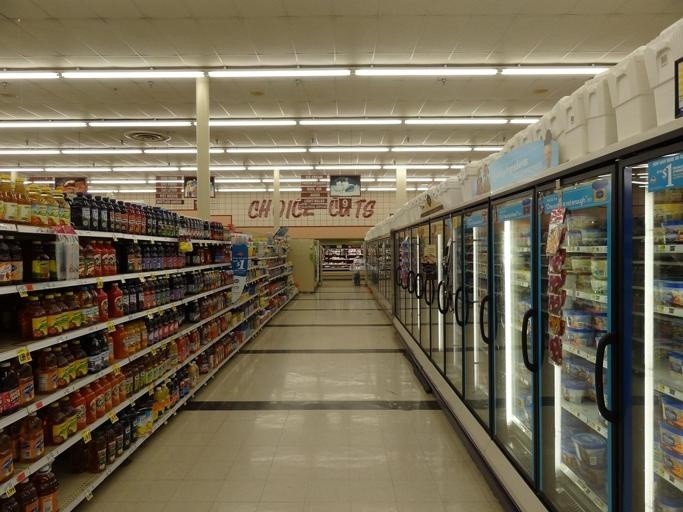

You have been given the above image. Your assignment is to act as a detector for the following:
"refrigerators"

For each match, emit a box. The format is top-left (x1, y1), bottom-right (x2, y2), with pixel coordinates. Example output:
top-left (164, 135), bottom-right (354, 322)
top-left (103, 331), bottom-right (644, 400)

top-left (395, 126), bottom-right (682, 511)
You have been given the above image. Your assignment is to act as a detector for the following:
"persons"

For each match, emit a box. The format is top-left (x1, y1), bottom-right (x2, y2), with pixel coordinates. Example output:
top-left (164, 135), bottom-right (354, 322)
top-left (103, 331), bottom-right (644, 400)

top-left (341, 177), bottom-right (349, 191)
top-left (349, 256), bottom-right (359, 286)
top-left (333, 180), bottom-right (340, 190)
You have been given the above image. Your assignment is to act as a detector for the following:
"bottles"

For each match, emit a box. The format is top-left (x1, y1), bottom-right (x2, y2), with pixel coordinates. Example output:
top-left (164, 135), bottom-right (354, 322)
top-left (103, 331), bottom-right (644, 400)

top-left (0, 170), bottom-right (249, 511)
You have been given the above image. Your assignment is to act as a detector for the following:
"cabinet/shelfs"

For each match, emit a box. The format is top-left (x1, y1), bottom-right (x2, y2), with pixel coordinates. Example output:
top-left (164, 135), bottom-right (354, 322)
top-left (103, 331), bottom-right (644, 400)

top-left (0, 222), bottom-right (300, 512)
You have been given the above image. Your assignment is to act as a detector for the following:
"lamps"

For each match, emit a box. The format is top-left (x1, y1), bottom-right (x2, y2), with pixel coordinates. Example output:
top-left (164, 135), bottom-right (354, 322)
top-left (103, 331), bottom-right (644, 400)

top-left (1, 164), bottom-right (465, 173)
top-left (2, 118), bottom-right (541, 131)
top-left (2, 64), bottom-right (617, 79)
top-left (2, 144), bottom-right (505, 155)
top-left (23, 178), bottom-right (448, 193)
